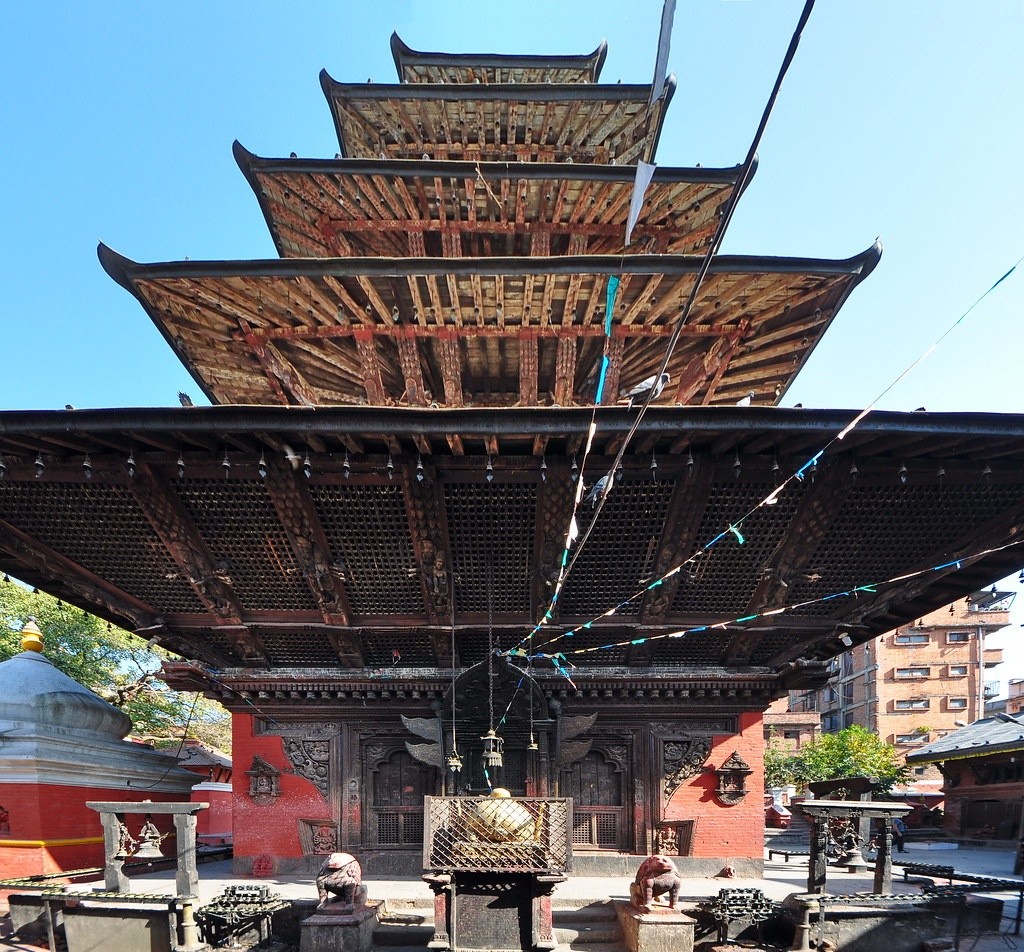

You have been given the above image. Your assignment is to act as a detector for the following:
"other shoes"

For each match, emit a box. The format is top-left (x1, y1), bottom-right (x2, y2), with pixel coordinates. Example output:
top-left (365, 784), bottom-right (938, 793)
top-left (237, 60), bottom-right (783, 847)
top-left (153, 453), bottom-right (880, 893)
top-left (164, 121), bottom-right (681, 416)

top-left (899, 850), bottom-right (905, 853)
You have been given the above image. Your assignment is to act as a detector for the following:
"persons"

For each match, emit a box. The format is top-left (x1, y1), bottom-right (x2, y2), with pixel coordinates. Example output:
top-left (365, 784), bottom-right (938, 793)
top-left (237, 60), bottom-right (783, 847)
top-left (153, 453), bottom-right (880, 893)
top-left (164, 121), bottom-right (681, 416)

top-left (892, 817), bottom-right (910, 852)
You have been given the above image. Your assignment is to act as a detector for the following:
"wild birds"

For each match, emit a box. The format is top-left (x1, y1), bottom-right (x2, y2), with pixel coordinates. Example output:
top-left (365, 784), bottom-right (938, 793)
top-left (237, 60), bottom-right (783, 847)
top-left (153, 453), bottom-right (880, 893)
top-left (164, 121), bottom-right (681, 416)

top-left (282, 444), bottom-right (302, 471)
top-left (583, 468), bottom-right (617, 508)
top-left (735, 390), bottom-right (755, 406)
top-left (622, 373), bottom-right (671, 412)
